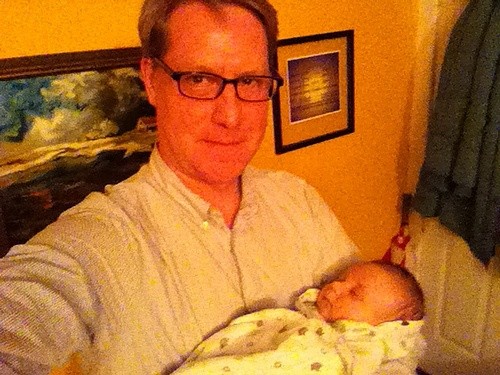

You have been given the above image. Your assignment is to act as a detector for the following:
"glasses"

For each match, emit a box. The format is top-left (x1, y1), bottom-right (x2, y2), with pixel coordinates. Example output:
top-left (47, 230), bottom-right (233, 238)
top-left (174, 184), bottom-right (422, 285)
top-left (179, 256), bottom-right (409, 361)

top-left (153, 57), bottom-right (284, 102)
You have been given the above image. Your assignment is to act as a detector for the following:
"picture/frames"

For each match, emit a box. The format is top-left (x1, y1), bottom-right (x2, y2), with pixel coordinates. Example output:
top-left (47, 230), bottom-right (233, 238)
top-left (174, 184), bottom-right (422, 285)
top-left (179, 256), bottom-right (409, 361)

top-left (272, 29), bottom-right (355, 156)
top-left (0, 46), bottom-right (157, 259)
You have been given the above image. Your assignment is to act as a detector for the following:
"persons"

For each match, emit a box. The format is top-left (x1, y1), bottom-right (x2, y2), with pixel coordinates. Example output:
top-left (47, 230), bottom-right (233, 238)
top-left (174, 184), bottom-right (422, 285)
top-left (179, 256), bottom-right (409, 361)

top-left (169, 259), bottom-right (426, 375)
top-left (0, 0), bottom-right (424, 375)
